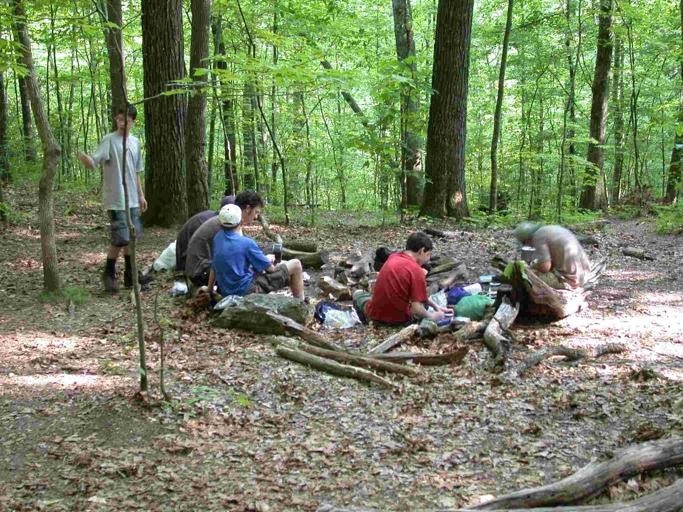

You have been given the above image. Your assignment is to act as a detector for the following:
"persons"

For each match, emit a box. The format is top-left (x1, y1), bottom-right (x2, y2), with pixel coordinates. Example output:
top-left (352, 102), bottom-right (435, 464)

top-left (352, 232), bottom-right (454, 327)
top-left (196, 204), bottom-right (305, 301)
top-left (176, 195), bottom-right (237, 271)
top-left (185, 190), bottom-right (264, 286)
top-left (513, 221), bottom-right (591, 291)
top-left (79, 104), bottom-right (154, 293)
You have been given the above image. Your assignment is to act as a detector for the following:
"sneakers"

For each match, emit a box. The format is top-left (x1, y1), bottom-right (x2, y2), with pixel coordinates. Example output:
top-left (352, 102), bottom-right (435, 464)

top-left (124, 270), bottom-right (155, 287)
top-left (103, 271), bottom-right (118, 291)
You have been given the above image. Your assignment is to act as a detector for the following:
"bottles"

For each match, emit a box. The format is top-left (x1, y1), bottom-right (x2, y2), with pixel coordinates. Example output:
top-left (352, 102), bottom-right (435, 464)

top-left (489, 283), bottom-right (501, 299)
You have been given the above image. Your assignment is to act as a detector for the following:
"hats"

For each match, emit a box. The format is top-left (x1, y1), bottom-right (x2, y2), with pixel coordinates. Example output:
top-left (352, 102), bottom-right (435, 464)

top-left (218, 203), bottom-right (242, 228)
top-left (512, 222), bottom-right (543, 241)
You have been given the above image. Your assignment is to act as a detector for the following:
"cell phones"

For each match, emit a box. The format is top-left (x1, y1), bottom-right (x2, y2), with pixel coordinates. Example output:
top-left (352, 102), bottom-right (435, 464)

top-left (272, 244), bottom-right (282, 252)
